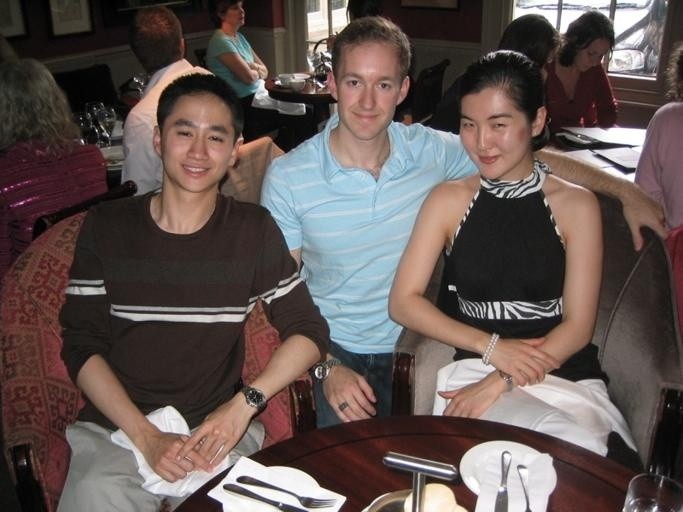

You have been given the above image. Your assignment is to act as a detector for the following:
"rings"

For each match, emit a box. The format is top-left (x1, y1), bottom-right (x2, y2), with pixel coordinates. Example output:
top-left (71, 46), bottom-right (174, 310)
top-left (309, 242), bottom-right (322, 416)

top-left (338, 402), bottom-right (347, 413)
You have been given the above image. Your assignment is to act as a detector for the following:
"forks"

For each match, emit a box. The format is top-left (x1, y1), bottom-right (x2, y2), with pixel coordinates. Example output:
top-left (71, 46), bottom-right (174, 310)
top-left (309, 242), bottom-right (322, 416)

top-left (236, 476), bottom-right (337, 509)
top-left (517, 465), bottom-right (532, 512)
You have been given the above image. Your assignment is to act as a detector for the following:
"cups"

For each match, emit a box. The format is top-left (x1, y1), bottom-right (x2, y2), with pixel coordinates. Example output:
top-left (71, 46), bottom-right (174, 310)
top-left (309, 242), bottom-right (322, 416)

top-left (622, 473), bottom-right (683, 512)
top-left (111, 121), bottom-right (123, 134)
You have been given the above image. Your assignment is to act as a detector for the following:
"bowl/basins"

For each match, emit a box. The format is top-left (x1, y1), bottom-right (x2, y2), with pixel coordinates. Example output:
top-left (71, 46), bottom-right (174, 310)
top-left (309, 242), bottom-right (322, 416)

top-left (278, 74), bottom-right (306, 90)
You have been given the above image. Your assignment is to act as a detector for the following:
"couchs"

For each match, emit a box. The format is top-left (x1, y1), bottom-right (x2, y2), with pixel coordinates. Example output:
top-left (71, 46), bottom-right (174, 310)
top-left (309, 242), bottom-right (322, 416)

top-left (0, 192), bottom-right (318, 512)
top-left (391, 184), bottom-right (681, 493)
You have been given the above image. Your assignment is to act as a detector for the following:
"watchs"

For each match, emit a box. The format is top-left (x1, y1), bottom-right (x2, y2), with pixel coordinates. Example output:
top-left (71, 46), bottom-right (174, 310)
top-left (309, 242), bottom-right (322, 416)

top-left (240, 383), bottom-right (268, 414)
top-left (309, 358), bottom-right (342, 382)
top-left (498, 370), bottom-right (514, 394)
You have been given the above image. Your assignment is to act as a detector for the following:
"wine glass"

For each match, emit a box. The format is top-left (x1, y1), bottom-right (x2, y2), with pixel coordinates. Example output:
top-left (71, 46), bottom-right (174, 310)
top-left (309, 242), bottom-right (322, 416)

top-left (73, 102), bottom-right (116, 150)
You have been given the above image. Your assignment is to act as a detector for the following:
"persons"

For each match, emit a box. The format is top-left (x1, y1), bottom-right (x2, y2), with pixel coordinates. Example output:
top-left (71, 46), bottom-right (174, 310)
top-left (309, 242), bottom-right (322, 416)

top-left (204, 0), bottom-right (310, 150)
top-left (635, 46), bottom-right (681, 234)
top-left (0, 58), bottom-right (108, 281)
top-left (119, 5), bottom-right (215, 198)
top-left (545, 11), bottom-right (618, 142)
top-left (56, 74), bottom-right (329, 512)
top-left (432, 14), bottom-right (561, 135)
top-left (388, 48), bottom-right (645, 473)
top-left (258, 14), bottom-right (669, 429)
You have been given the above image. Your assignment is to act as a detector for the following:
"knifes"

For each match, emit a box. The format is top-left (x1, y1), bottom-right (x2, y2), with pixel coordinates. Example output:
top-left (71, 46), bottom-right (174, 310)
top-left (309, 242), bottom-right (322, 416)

top-left (223, 484), bottom-right (309, 512)
top-left (494, 450), bottom-right (512, 512)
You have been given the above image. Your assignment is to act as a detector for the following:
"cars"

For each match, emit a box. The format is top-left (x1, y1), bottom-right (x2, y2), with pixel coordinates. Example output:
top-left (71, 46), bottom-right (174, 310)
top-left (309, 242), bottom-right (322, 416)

top-left (513, 0), bottom-right (668, 78)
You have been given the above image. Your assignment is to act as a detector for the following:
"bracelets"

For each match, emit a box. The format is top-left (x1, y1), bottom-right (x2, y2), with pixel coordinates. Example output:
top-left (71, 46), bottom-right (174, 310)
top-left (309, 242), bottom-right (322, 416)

top-left (482, 331), bottom-right (498, 367)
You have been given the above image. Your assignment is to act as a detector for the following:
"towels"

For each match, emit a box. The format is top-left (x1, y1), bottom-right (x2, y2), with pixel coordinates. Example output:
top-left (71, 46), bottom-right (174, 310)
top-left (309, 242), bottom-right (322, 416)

top-left (430, 355), bottom-right (639, 456)
top-left (109, 403), bottom-right (232, 498)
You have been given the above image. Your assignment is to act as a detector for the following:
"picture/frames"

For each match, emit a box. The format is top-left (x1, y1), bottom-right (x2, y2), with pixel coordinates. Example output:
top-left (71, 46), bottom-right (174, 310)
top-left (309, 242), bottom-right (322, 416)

top-left (0, 0), bottom-right (29, 39)
top-left (43, 0), bottom-right (96, 41)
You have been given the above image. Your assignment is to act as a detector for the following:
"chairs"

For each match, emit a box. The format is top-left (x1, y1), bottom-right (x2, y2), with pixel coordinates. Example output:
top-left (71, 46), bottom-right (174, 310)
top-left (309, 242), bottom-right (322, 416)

top-left (394, 59), bottom-right (451, 124)
top-left (33, 179), bottom-right (138, 240)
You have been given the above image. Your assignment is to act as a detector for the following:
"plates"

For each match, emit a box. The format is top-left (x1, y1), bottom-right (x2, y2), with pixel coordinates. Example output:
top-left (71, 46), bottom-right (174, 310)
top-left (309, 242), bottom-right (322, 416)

top-left (102, 130), bottom-right (125, 139)
top-left (99, 145), bottom-right (123, 168)
top-left (274, 73), bottom-right (311, 86)
top-left (460, 441), bottom-right (558, 498)
top-left (222, 466), bottom-right (319, 512)
